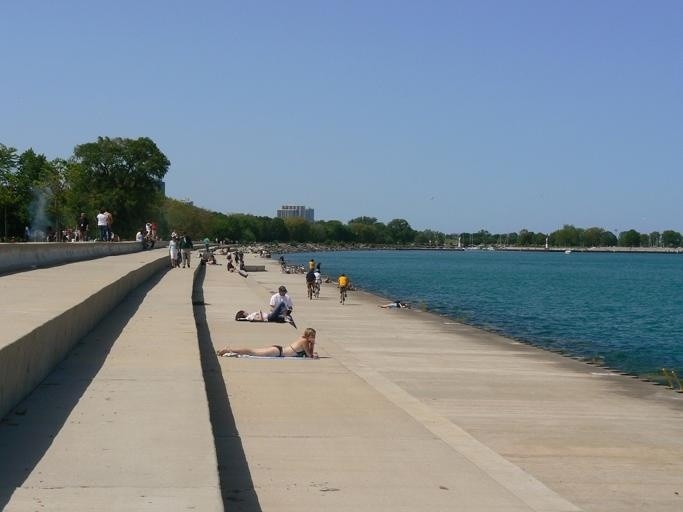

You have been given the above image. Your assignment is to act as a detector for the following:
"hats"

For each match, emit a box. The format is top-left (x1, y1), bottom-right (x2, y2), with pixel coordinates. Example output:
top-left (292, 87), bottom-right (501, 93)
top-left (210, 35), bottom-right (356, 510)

top-left (279, 285), bottom-right (287, 292)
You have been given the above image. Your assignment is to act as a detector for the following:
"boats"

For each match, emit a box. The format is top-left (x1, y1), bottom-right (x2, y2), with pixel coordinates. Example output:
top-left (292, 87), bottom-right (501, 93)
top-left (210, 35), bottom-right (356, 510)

top-left (564, 249), bottom-right (571, 255)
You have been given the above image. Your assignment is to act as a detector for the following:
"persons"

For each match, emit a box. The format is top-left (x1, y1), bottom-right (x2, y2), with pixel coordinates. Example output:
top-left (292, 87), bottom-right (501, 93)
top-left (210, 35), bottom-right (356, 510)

top-left (336, 272), bottom-right (350, 305)
top-left (60, 227), bottom-right (79, 244)
top-left (306, 259), bottom-right (322, 298)
top-left (269, 285), bottom-right (292, 316)
top-left (134, 221), bottom-right (157, 242)
top-left (203, 237), bottom-right (245, 272)
top-left (215, 327), bottom-right (318, 359)
top-left (96, 209), bottom-right (108, 243)
top-left (379, 301), bottom-right (412, 309)
top-left (235, 302), bottom-right (293, 323)
top-left (79, 212), bottom-right (88, 243)
top-left (103, 207), bottom-right (113, 242)
top-left (177, 232), bottom-right (193, 270)
top-left (167, 231), bottom-right (178, 270)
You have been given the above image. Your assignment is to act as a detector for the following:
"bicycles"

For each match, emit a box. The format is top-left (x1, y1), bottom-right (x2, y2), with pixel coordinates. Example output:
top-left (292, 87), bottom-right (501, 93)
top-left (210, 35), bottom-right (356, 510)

top-left (306, 281), bottom-right (322, 300)
top-left (337, 287), bottom-right (350, 305)
